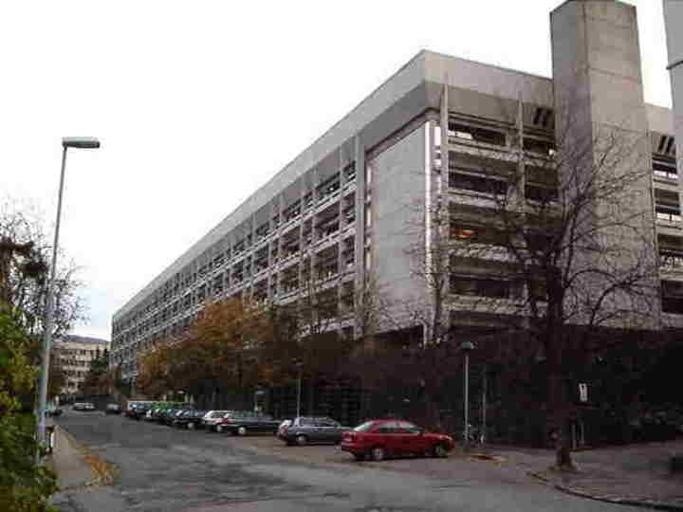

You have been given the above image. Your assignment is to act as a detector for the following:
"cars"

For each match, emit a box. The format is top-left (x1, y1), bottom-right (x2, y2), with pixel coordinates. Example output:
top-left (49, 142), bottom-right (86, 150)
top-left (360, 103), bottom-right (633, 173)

top-left (340, 416), bottom-right (456, 462)
top-left (73, 400), bottom-right (282, 437)
top-left (278, 415), bottom-right (354, 446)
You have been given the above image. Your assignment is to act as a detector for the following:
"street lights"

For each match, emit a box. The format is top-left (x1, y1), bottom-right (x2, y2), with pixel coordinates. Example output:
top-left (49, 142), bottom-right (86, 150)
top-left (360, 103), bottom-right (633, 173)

top-left (35, 136), bottom-right (99, 448)
